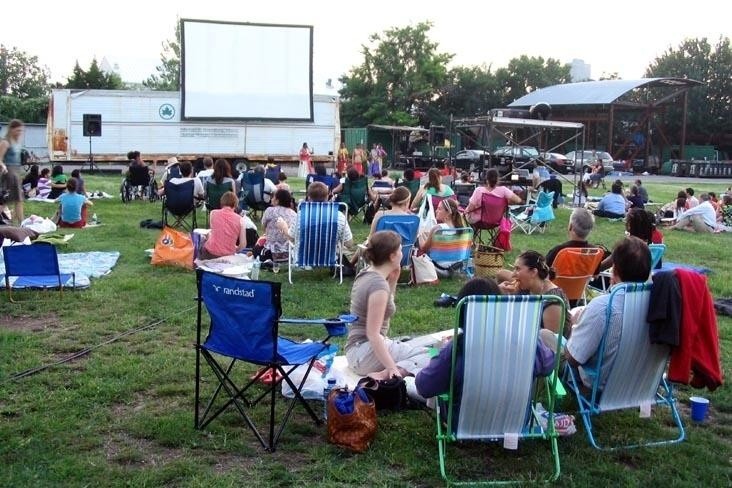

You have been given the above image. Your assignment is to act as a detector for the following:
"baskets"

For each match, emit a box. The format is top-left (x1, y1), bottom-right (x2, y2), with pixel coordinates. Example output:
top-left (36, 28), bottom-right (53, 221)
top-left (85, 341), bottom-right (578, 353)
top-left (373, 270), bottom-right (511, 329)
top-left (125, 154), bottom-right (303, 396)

top-left (472, 244), bottom-right (504, 279)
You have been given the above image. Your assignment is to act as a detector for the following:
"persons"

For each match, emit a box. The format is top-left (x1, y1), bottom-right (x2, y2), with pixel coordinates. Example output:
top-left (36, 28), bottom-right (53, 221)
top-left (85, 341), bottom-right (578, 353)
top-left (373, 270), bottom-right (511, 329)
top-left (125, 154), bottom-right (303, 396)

top-left (645, 210), bottom-right (662, 245)
top-left (0, 193), bottom-right (11, 246)
top-left (130, 151), bottom-right (156, 179)
top-left (414, 169), bottom-right (424, 181)
top-left (0, 119), bottom-right (25, 225)
top-left (544, 208), bottom-right (600, 308)
top-left (601, 207), bottom-right (662, 299)
top-left (345, 230), bottom-right (438, 382)
top-left (233, 206), bottom-right (256, 231)
top-left (708, 195), bottom-right (724, 225)
top-left (587, 149), bottom-right (598, 174)
top-left (377, 145), bottom-right (388, 177)
top-left (21, 165), bottom-right (39, 201)
top-left (372, 173), bottom-right (385, 191)
top-left (382, 170), bottom-right (393, 189)
top-left (418, 198), bottom-right (468, 278)
top-left (582, 160), bottom-right (597, 184)
top-left (614, 180), bottom-right (625, 198)
top-left (298, 142), bottom-right (314, 181)
top-left (333, 169), bottom-right (377, 212)
top-left (726, 187), bottom-right (732, 197)
top-left (352, 142), bottom-right (365, 178)
top-left (415, 278), bottom-right (555, 432)
top-left (266, 158), bottom-right (282, 185)
top-left (200, 192), bottom-right (247, 261)
top-left (570, 182), bottom-right (589, 207)
top-left (669, 194), bottom-right (717, 234)
top-left (454, 171), bottom-right (472, 186)
top-left (529, 157), bottom-right (550, 192)
top-left (499, 251), bottom-right (570, 373)
top-left (626, 186), bottom-right (644, 212)
top-left (153, 161), bottom-right (204, 206)
top-left (160, 157), bottom-right (182, 187)
top-left (412, 169), bottom-right (459, 214)
top-left (308, 164), bottom-right (336, 193)
top-left (337, 142), bottom-right (349, 178)
top-left (589, 184), bottom-right (625, 218)
top-left (50, 165), bottom-right (67, 197)
top-left (350, 187), bottom-right (420, 265)
top-left (370, 142), bottom-right (380, 177)
top-left (398, 169), bottom-right (420, 208)
top-left (198, 158), bottom-right (214, 190)
top-left (720, 196), bottom-right (732, 227)
top-left (553, 237), bottom-right (653, 413)
top-left (121, 153), bottom-right (134, 179)
top-left (72, 168), bottom-right (88, 199)
top-left (275, 180), bottom-right (354, 265)
top-left (635, 179), bottom-right (648, 204)
top-left (673, 199), bottom-right (688, 222)
top-left (685, 188), bottom-right (699, 211)
top-left (660, 191), bottom-right (687, 215)
top-left (204, 159), bottom-right (237, 213)
top-left (589, 159), bottom-right (604, 187)
top-left (463, 168), bottom-right (523, 230)
top-left (242, 165), bottom-right (277, 206)
top-left (273, 173), bottom-right (293, 197)
top-left (256, 189), bottom-right (298, 266)
top-left (707, 191), bottom-right (718, 204)
top-left (55, 179), bottom-right (93, 229)
top-left (225, 156), bottom-right (243, 199)
top-left (37, 168), bottom-right (68, 200)
top-left (434, 160), bottom-right (457, 184)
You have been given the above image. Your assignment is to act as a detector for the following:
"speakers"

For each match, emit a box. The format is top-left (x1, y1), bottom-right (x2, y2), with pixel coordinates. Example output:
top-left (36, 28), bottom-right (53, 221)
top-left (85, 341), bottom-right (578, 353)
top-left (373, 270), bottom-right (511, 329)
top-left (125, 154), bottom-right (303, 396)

top-left (428, 125), bottom-right (445, 146)
top-left (83, 113), bottom-right (102, 137)
top-left (229, 157), bottom-right (251, 179)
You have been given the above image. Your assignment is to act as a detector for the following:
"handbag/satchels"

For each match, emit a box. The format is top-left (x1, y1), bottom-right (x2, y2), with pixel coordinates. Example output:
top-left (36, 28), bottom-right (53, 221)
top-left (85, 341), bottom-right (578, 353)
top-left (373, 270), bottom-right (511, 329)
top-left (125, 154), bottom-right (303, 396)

top-left (326, 388), bottom-right (378, 454)
top-left (410, 254), bottom-right (440, 286)
top-left (150, 226), bottom-right (195, 270)
top-left (363, 193), bottom-right (383, 226)
top-left (21, 149), bottom-right (30, 166)
top-left (493, 215), bottom-right (512, 251)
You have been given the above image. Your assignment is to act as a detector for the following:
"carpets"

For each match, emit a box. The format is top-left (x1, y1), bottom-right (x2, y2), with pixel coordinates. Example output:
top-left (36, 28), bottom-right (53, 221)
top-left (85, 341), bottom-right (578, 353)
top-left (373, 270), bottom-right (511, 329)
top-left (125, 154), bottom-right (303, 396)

top-left (28, 192), bottom-right (115, 202)
top-left (0, 250), bottom-right (120, 288)
top-left (281, 327), bottom-right (463, 400)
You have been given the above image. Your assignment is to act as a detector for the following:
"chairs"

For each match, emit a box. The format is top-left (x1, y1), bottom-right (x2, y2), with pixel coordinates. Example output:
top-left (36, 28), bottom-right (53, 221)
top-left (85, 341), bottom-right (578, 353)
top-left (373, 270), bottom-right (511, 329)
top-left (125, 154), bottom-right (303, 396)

top-left (265, 165), bottom-right (281, 184)
top-left (241, 172), bottom-right (271, 218)
top-left (162, 180), bottom-right (197, 231)
top-left (357, 215), bottom-right (421, 286)
top-left (429, 227), bottom-right (473, 276)
top-left (342, 177), bottom-right (368, 223)
top-left (193, 267), bottom-right (360, 454)
top-left (436, 293), bottom-right (565, 485)
top-left (398, 178), bottom-right (420, 206)
top-left (563, 282), bottom-right (685, 451)
top-left (451, 180), bottom-right (476, 206)
top-left (509, 191), bottom-right (556, 236)
top-left (428, 195), bottom-right (464, 222)
top-left (306, 174), bottom-right (333, 198)
top-left (465, 195), bottom-right (506, 249)
top-left (205, 181), bottom-right (233, 227)
top-left (288, 202), bottom-right (348, 285)
top-left (3, 244), bottom-right (75, 303)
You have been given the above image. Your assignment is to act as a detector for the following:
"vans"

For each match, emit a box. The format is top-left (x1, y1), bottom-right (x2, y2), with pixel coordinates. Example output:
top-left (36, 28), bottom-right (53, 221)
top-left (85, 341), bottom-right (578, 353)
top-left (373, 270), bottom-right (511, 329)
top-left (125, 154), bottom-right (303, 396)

top-left (491, 146), bottom-right (545, 173)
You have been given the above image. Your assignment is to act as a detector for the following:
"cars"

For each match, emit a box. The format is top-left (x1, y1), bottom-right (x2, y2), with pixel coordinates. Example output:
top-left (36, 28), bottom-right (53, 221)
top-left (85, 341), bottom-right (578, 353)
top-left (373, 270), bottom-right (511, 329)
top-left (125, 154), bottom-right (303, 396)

top-left (450, 150), bottom-right (492, 172)
top-left (540, 153), bottom-right (575, 175)
top-left (564, 151), bottom-right (615, 176)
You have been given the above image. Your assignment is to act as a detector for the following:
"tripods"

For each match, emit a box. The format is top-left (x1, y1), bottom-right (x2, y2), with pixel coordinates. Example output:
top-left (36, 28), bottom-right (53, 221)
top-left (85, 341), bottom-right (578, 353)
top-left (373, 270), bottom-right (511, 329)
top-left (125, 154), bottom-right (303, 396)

top-left (80, 136), bottom-right (104, 177)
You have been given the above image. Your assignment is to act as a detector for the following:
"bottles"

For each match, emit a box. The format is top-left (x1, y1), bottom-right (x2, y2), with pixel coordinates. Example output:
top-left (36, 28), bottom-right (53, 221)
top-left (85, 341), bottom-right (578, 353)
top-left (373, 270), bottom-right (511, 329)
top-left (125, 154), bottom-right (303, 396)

top-left (323, 378), bottom-right (340, 426)
top-left (251, 255), bottom-right (261, 280)
top-left (466, 257), bottom-right (474, 279)
top-left (92, 213), bottom-right (97, 226)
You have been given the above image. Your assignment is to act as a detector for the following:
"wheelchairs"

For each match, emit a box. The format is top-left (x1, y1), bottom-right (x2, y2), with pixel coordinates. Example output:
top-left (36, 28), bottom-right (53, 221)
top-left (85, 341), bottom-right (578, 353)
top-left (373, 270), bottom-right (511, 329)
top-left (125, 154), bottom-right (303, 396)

top-left (119, 162), bottom-right (160, 204)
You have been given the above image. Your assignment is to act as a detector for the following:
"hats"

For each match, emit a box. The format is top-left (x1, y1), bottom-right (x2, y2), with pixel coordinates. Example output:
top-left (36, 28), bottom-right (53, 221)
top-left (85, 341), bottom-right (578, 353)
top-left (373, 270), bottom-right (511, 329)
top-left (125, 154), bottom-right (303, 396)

top-left (166, 157), bottom-right (180, 169)
